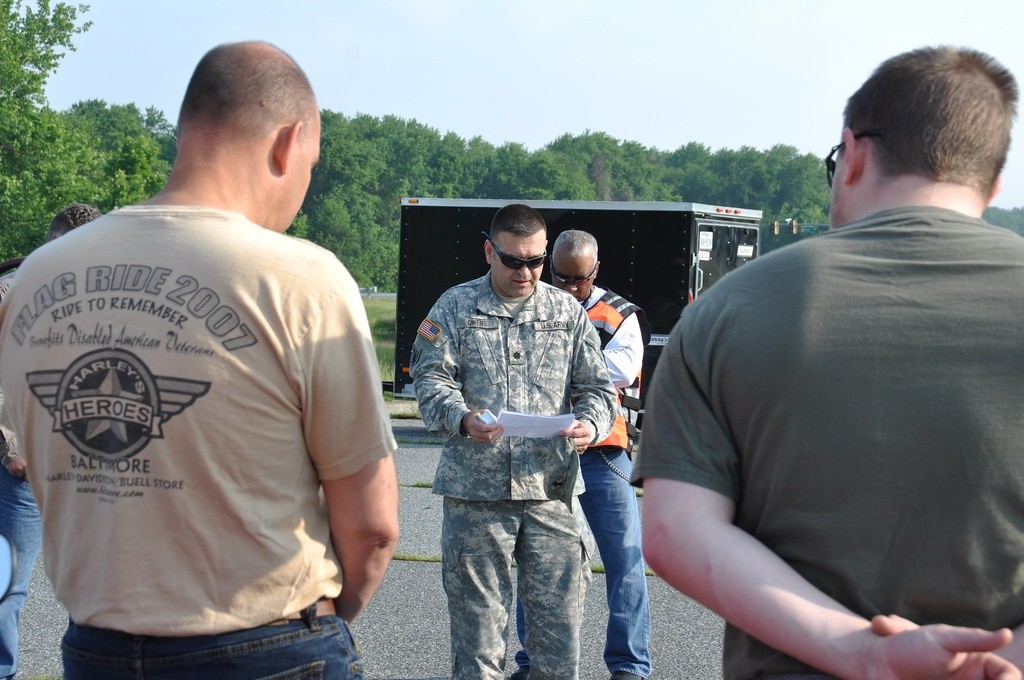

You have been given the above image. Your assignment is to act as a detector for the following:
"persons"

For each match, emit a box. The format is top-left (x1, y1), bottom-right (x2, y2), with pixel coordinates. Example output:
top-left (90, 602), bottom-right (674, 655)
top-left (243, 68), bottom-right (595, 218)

top-left (0, 205), bottom-right (104, 680)
top-left (510, 230), bottom-right (652, 680)
top-left (409, 204), bottom-right (618, 680)
top-left (0, 42), bottom-right (400, 680)
top-left (629, 49), bottom-right (1024, 678)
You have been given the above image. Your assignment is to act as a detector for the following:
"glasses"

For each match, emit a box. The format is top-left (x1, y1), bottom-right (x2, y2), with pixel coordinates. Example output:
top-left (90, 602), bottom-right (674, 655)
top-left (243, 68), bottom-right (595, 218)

top-left (480, 231), bottom-right (547, 269)
top-left (824, 129), bottom-right (886, 189)
top-left (549, 261), bottom-right (599, 287)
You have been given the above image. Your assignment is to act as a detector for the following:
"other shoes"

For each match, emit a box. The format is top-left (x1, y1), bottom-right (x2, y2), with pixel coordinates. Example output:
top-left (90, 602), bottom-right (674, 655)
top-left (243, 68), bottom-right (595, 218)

top-left (610, 670), bottom-right (644, 679)
top-left (511, 666), bottom-right (530, 679)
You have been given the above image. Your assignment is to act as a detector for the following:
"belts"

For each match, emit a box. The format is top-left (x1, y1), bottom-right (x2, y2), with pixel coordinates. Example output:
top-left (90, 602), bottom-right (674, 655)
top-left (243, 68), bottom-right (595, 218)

top-left (278, 597), bottom-right (337, 620)
